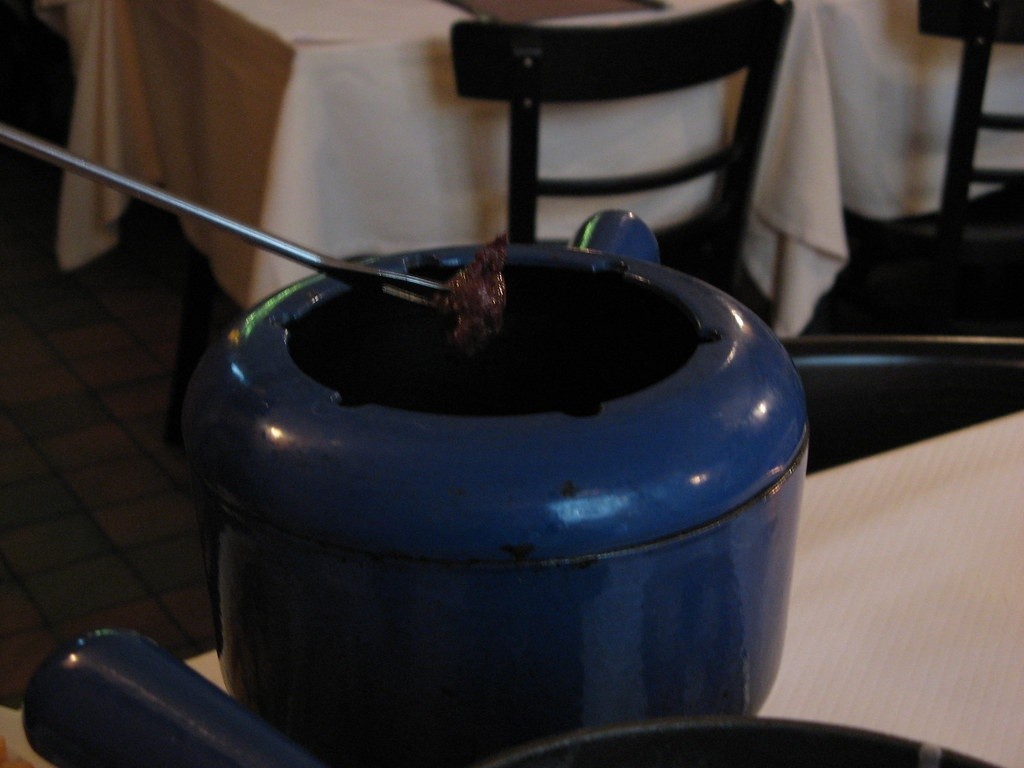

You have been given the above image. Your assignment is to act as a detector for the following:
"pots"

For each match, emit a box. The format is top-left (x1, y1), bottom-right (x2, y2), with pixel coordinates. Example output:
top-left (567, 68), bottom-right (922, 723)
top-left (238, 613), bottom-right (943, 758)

top-left (24, 627), bottom-right (1006, 767)
top-left (181, 209), bottom-right (812, 730)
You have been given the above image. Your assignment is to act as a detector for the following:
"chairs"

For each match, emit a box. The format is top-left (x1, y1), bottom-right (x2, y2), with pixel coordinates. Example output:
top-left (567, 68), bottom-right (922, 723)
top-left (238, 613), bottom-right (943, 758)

top-left (451, 0), bottom-right (793, 295)
top-left (843, 0), bottom-right (1024, 330)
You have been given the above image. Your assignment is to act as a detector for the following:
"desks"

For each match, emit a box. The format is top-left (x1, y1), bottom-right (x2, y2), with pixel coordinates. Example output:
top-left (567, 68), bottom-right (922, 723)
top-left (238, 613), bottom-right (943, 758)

top-left (36, 0), bottom-right (1024, 447)
top-left (0, 411), bottom-right (1024, 768)
